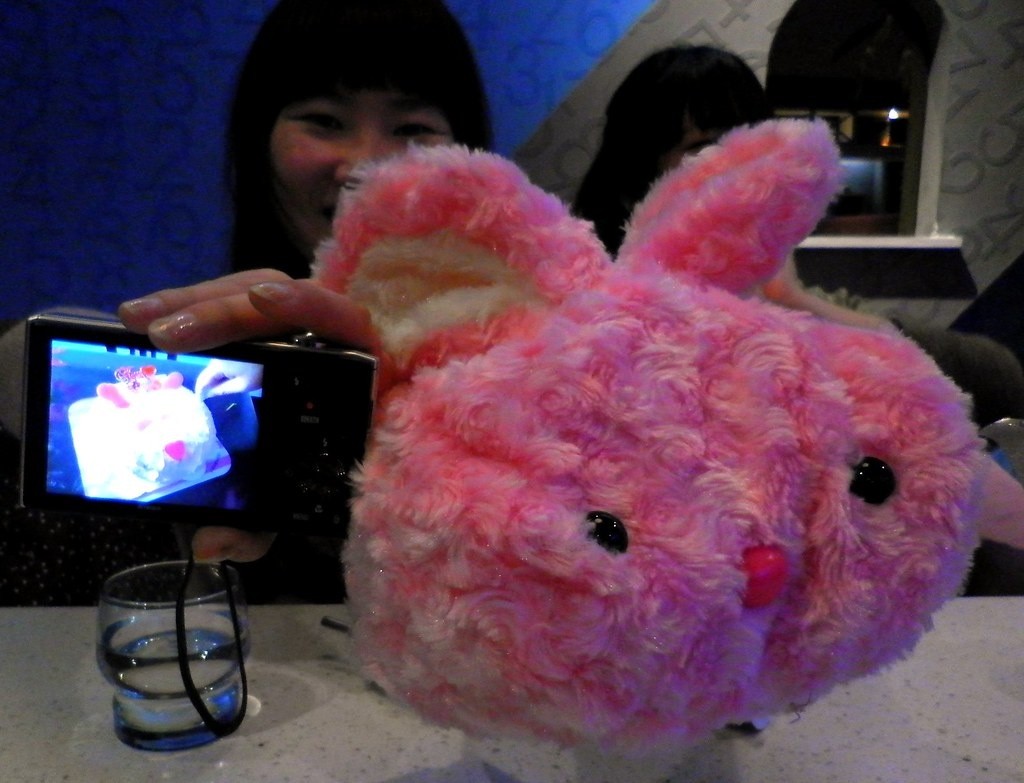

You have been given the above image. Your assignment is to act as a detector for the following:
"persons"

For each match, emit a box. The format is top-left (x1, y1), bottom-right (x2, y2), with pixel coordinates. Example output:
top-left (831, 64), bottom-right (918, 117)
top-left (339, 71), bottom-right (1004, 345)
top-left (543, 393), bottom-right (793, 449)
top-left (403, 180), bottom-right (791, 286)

top-left (571, 44), bottom-right (1024, 421)
top-left (119, 0), bottom-right (493, 565)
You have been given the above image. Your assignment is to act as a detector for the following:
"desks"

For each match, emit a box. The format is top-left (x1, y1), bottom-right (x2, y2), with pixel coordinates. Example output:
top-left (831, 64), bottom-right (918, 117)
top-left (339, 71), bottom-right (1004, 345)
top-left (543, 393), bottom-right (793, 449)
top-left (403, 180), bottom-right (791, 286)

top-left (0, 595), bottom-right (1024, 783)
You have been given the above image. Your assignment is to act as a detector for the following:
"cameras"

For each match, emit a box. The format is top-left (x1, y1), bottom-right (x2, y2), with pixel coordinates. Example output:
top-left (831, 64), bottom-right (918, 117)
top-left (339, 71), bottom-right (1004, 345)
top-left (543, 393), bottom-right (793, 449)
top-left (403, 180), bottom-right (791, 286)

top-left (19, 314), bottom-right (378, 550)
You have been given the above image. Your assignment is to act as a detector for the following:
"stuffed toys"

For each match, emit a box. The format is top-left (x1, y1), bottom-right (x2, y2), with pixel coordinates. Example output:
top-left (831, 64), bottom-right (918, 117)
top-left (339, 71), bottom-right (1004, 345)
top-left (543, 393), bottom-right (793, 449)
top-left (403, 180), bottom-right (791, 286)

top-left (309, 117), bottom-right (991, 757)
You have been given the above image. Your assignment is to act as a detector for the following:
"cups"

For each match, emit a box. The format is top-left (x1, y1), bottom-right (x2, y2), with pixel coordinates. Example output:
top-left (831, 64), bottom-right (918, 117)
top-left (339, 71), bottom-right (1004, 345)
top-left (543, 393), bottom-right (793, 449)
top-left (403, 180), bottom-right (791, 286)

top-left (96, 560), bottom-right (251, 752)
